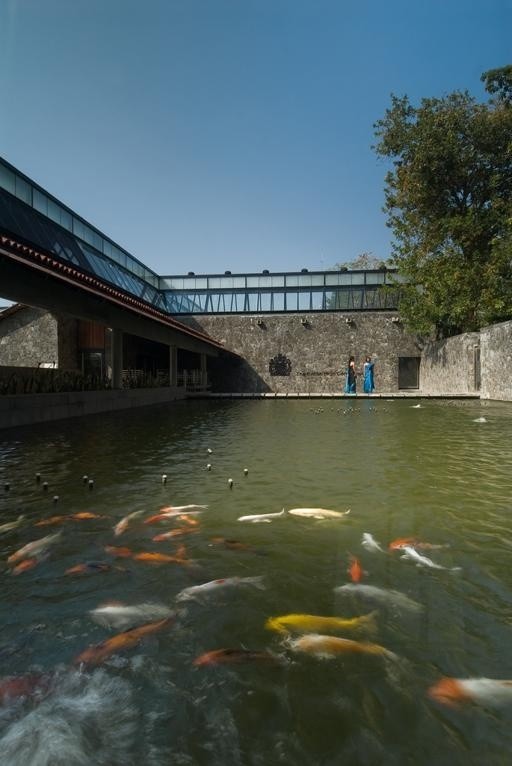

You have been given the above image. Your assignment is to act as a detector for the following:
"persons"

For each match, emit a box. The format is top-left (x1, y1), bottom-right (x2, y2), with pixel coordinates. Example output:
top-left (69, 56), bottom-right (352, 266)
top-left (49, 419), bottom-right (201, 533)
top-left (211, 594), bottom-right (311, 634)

top-left (344, 356), bottom-right (358, 395)
top-left (362, 357), bottom-right (375, 394)
